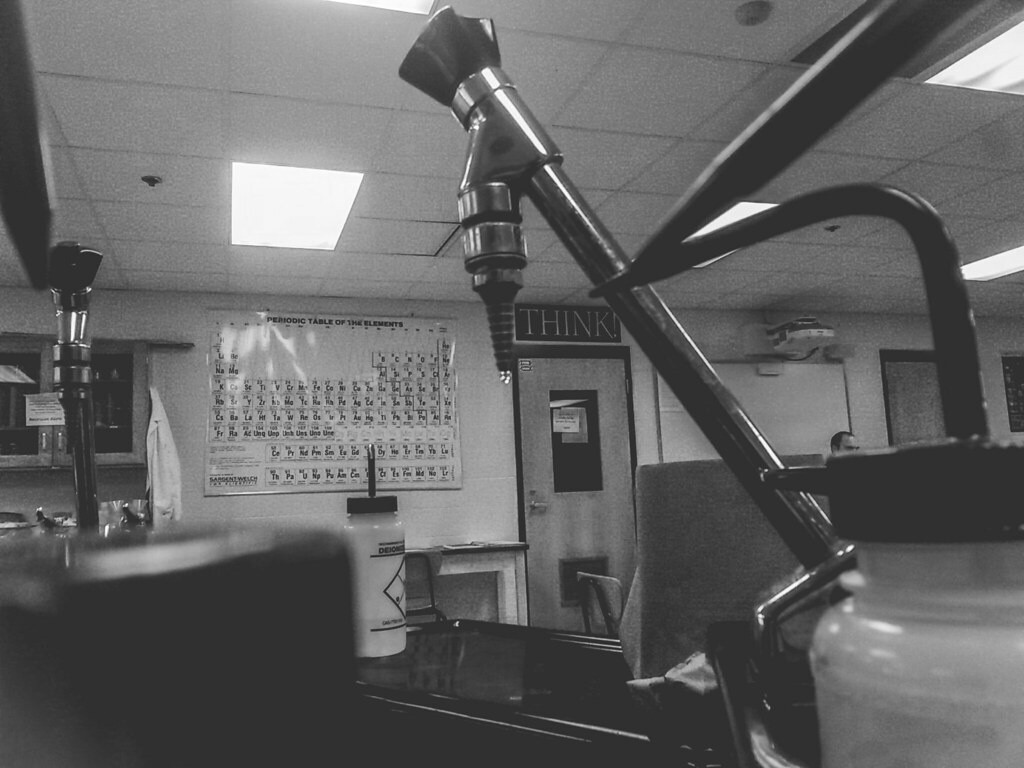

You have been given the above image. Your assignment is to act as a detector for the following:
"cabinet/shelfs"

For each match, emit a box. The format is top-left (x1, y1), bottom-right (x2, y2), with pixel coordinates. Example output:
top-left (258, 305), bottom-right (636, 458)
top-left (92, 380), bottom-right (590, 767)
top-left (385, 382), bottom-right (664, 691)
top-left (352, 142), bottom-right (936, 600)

top-left (1, 331), bottom-right (150, 471)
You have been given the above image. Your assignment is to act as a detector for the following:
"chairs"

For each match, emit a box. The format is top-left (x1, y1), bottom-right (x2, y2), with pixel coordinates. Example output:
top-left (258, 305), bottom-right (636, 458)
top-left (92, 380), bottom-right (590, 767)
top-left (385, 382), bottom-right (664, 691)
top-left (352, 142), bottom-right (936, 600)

top-left (576, 571), bottom-right (625, 639)
top-left (405, 550), bottom-right (449, 624)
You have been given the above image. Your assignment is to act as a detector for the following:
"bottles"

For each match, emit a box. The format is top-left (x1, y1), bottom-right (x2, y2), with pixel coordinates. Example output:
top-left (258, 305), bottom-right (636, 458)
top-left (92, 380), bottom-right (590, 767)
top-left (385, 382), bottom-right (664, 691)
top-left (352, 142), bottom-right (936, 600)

top-left (341, 493), bottom-right (406, 658)
top-left (808, 439), bottom-right (1024, 768)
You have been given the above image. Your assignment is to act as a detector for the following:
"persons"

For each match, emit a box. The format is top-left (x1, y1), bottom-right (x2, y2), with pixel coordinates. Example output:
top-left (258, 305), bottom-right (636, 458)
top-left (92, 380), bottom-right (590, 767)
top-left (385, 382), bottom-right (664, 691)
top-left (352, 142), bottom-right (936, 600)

top-left (830, 431), bottom-right (860, 452)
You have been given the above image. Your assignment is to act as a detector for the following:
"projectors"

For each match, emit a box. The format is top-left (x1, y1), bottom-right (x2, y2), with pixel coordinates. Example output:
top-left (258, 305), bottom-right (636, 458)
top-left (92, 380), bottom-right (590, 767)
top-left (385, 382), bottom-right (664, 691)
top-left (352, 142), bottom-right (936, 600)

top-left (774, 321), bottom-right (835, 354)
